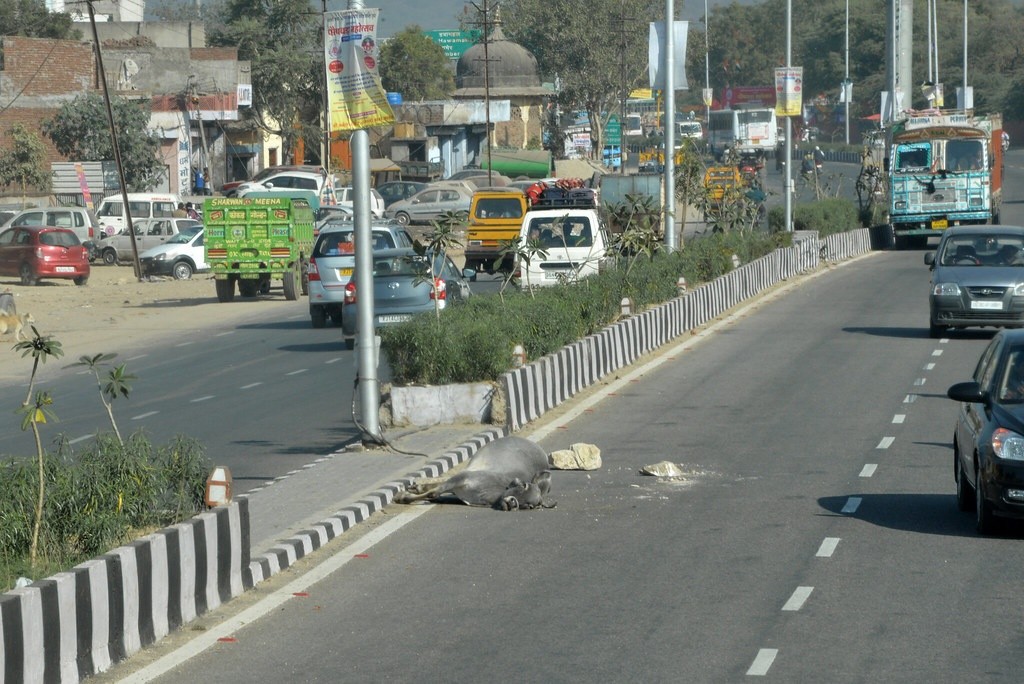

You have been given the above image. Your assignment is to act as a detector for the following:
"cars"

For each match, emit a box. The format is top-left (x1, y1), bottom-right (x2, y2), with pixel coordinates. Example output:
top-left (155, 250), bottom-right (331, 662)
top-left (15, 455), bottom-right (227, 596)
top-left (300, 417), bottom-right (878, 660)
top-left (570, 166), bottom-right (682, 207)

top-left (341, 246), bottom-right (472, 344)
top-left (382, 188), bottom-right (471, 226)
top-left (308, 223), bottom-right (422, 329)
top-left (94, 194), bottom-right (184, 236)
top-left (221, 165), bottom-right (387, 223)
top-left (96, 217), bottom-right (202, 267)
top-left (132, 226), bottom-right (213, 280)
top-left (622, 113), bottom-right (745, 223)
top-left (375, 169), bottom-right (597, 213)
top-left (947, 327), bottom-right (1024, 531)
top-left (0, 205), bottom-right (97, 263)
top-left (514, 207), bottom-right (616, 292)
top-left (924, 224), bottom-right (1024, 336)
top-left (0, 224), bottom-right (90, 284)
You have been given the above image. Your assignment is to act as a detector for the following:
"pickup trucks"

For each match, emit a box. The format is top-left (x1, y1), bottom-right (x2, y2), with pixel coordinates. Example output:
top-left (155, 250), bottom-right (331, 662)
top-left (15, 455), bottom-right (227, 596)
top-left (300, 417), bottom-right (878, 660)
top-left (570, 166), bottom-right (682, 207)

top-left (463, 188), bottom-right (530, 280)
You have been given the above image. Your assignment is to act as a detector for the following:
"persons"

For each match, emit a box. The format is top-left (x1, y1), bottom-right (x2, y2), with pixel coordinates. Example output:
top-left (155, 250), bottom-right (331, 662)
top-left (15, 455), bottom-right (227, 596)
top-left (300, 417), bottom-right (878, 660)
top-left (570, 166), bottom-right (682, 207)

top-left (776, 142), bottom-right (785, 169)
top-left (173, 203), bottom-right (187, 219)
top-left (204, 168), bottom-right (210, 196)
top-left (996, 244), bottom-right (1022, 265)
top-left (802, 152), bottom-right (814, 171)
top-left (968, 149), bottom-right (981, 170)
top-left (756, 149), bottom-right (768, 160)
top-left (814, 146), bottom-right (823, 164)
top-left (185, 203), bottom-right (198, 220)
top-left (739, 156), bottom-right (756, 170)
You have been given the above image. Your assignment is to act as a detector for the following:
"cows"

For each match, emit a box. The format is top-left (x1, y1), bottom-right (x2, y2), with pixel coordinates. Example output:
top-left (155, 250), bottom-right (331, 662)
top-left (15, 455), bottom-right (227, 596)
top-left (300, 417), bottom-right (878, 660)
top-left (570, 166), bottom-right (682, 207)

top-left (393, 433), bottom-right (557, 511)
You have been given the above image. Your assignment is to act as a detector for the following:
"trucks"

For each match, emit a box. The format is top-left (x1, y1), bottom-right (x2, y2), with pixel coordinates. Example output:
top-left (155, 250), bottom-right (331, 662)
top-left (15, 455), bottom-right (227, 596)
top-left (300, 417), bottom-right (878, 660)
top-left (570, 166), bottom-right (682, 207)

top-left (855, 109), bottom-right (1003, 248)
top-left (599, 173), bottom-right (661, 252)
top-left (202, 190), bottom-right (321, 301)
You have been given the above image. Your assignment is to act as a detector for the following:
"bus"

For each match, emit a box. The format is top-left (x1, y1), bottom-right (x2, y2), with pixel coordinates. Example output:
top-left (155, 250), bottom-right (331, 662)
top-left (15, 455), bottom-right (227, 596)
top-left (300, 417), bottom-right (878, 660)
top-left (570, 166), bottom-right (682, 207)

top-left (708, 108), bottom-right (778, 156)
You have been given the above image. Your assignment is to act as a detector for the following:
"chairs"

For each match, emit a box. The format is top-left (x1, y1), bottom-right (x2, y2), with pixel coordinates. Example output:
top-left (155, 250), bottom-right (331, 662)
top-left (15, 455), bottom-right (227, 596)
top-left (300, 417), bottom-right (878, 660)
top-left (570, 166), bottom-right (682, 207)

top-left (42, 235), bottom-right (55, 245)
top-left (152, 224), bottom-right (161, 235)
top-left (22, 236), bottom-right (29, 244)
top-left (376, 262), bottom-right (392, 275)
top-left (410, 261), bottom-right (423, 270)
top-left (547, 228), bottom-right (588, 248)
top-left (377, 237), bottom-right (387, 249)
top-left (330, 237), bottom-right (345, 250)
top-left (953, 247), bottom-right (1023, 265)
